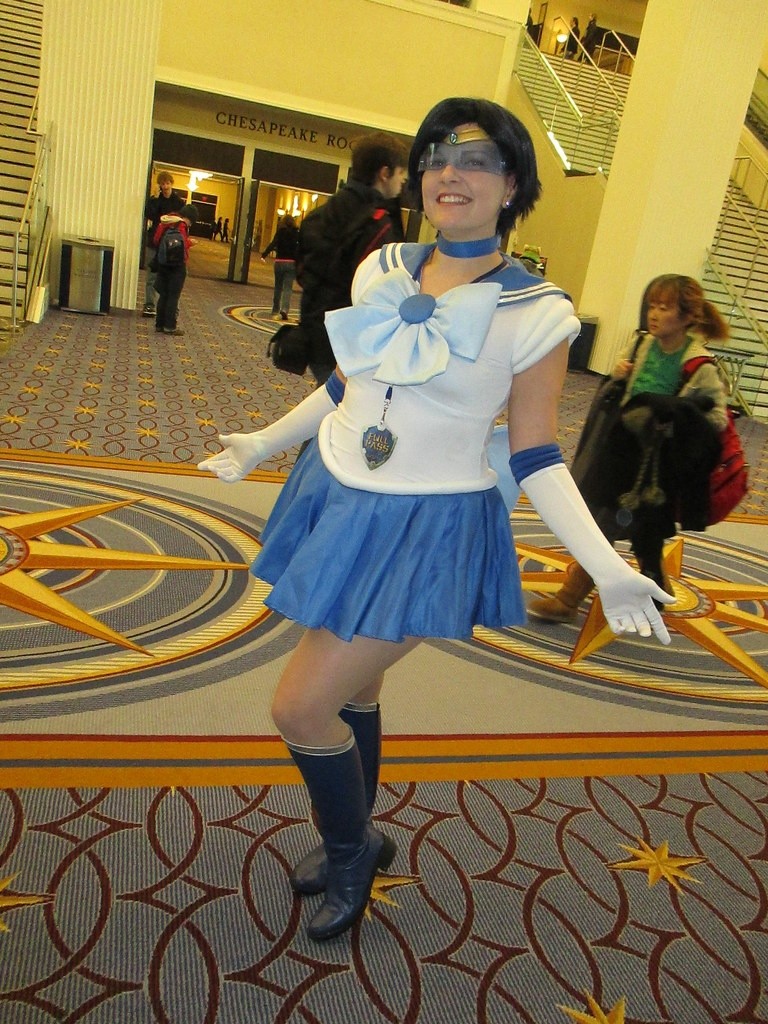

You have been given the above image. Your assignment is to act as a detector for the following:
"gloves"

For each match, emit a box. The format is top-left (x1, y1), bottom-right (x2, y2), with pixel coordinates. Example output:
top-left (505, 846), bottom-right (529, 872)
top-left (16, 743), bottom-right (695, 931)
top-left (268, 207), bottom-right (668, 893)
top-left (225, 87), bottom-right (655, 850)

top-left (197, 368), bottom-right (345, 484)
top-left (509, 443), bottom-right (677, 645)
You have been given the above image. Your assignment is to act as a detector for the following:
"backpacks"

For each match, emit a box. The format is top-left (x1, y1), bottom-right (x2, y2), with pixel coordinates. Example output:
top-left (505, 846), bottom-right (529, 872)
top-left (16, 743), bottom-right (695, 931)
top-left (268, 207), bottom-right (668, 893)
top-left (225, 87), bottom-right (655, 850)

top-left (158, 221), bottom-right (185, 266)
top-left (681, 355), bottom-right (750, 526)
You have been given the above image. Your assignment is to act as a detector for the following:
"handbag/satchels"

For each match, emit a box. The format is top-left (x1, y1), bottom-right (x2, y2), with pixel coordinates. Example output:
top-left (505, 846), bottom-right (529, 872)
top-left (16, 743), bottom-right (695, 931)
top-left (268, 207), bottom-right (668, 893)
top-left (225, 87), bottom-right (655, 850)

top-left (593, 334), bottom-right (644, 411)
top-left (266, 324), bottom-right (313, 376)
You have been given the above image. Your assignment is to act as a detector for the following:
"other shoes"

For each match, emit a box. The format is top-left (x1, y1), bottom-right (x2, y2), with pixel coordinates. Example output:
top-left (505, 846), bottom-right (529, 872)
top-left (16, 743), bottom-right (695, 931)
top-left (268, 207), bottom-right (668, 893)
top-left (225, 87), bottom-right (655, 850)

top-left (156, 326), bottom-right (185, 336)
top-left (142, 306), bottom-right (157, 316)
top-left (272, 307), bottom-right (288, 320)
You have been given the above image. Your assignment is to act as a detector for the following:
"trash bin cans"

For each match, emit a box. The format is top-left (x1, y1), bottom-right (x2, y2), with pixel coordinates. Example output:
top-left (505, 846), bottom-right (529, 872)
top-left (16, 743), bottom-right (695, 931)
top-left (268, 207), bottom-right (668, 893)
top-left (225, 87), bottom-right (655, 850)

top-left (568, 312), bottom-right (599, 373)
top-left (59, 234), bottom-right (115, 317)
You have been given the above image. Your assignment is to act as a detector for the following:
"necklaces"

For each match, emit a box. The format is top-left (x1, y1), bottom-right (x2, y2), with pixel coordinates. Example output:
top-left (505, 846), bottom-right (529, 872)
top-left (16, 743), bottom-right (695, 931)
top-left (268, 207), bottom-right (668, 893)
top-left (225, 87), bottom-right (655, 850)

top-left (360, 248), bottom-right (508, 470)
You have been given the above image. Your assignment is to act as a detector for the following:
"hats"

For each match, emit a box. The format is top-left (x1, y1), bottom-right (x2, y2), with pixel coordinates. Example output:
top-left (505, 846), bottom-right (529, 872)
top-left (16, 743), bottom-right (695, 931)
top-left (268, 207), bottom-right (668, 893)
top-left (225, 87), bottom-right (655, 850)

top-left (178, 204), bottom-right (199, 224)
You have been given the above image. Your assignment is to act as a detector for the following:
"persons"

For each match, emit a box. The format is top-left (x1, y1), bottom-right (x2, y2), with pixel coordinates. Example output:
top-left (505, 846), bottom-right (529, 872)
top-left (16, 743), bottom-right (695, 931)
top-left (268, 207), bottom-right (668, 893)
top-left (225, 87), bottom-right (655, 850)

top-left (559, 17), bottom-right (580, 60)
top-left (151, 203), bottom-right (199, 335)
top-left (517, 244), bottom-right (543, 278)
top-left (296, 132), bottom-right (411, 390)
top-left (578, 13), bottom-right (598, 66)
top-left (220, 218), bottom-right (229, 243)
top-left (259, 215), bottom-right (298, 320)
top-left (526, 274), bottom-right (731, 626)
top-left (214, 217), bottom-right (223, 241)
top-left (198, 97), bottom-right (675, 943)
top-left (140, 171), bottom-right (185, 315)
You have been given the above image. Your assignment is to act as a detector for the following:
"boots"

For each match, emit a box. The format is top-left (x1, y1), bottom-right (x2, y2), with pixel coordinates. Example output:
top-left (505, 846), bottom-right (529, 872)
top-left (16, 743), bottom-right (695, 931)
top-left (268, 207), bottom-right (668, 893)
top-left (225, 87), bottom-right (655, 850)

top-left (289, 702), bottom-right (382, 896)
top-left (281, 723), bottom-right (398, 943)
top-left (527, 562), bottom-right (595, 622)
top-left (631, 545), bottom-right (666, 611)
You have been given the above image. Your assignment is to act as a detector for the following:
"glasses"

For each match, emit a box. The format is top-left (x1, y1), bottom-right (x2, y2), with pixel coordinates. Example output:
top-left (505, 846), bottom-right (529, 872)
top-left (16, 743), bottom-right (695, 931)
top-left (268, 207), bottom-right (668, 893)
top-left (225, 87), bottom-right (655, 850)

top-left (417, 141), bottom-right (508, 176)
